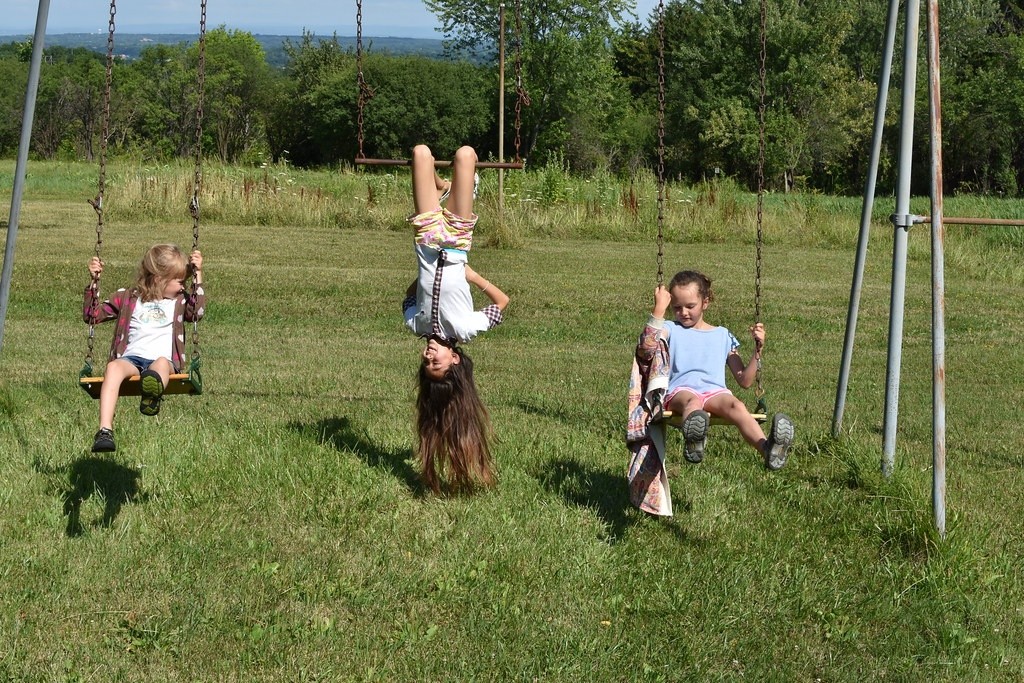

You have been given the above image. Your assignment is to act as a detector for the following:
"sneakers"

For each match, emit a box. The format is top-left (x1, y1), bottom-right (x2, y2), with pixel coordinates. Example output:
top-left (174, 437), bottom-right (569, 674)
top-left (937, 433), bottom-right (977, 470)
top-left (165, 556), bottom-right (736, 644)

top-left (91, 428), bottom-right (116, 452)
top-left (140, 370), bottom-right (164, 416)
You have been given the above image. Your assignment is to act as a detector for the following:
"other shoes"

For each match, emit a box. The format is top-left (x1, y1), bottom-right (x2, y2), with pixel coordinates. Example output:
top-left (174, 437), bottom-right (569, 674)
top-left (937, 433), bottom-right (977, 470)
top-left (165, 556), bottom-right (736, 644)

top-left (471, 172), bottom-right (478, 199)
top-left (682, 409), bottom-right (709, 463)
top-left (439, 182), bottom-right (452, 204)
top-left (763, 414), bottom-right (794, 471)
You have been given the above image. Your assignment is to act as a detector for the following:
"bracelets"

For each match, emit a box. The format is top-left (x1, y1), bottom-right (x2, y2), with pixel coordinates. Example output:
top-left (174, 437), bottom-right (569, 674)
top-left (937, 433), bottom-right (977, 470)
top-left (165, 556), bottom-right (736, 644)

top-left (482, 280), bottom-right (490, 292)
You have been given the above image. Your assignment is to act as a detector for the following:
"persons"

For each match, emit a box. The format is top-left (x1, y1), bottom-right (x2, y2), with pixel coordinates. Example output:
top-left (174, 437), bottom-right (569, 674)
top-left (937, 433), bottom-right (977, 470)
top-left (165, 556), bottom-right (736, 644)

top-left (403, 145), bottom-right (511, 495)
top-left (87, 243), bottom-right (204, 451)
top-left (637, 271), bottom-right (795, 470)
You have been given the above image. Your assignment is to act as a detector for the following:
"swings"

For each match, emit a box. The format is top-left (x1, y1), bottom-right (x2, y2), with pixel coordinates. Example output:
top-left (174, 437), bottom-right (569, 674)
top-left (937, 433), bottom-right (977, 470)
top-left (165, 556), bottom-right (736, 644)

top-left (354, 0), bottom-right (525, 169)
top-left (77, 0), bottom-right (206, 400)
top-left (653, 0), bottom-right (772, 426)
